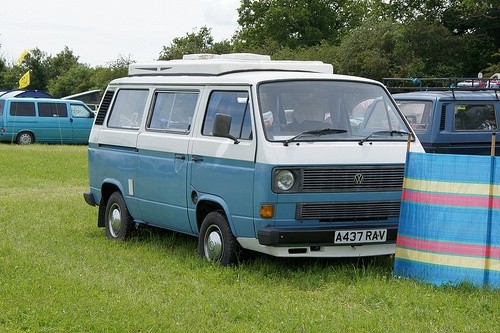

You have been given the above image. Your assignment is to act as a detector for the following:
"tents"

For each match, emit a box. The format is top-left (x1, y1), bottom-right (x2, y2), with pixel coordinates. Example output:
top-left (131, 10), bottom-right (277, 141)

top-left (0, 90), bottom-right (53, 98)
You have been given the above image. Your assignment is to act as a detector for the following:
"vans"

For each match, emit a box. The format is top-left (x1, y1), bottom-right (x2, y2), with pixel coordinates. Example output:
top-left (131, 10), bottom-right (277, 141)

top-left (356, 74), bottom-right (500, 154)
top-left (0, 98), bottom-right (97, 145)
top-left (82, 53), bottom-right (426, 270)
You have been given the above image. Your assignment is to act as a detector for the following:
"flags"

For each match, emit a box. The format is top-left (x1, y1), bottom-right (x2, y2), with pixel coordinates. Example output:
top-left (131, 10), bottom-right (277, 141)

top-left (18, 49), bottom-right (28, 65)
top-left (18, 70), bottom-right (31, 89)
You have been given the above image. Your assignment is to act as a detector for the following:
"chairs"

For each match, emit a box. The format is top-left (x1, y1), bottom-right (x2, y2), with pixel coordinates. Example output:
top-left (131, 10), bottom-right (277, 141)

top-left (455, 103), bottom-right (489, 130)
top-left (294, 103), bottom-right (325, 132)
